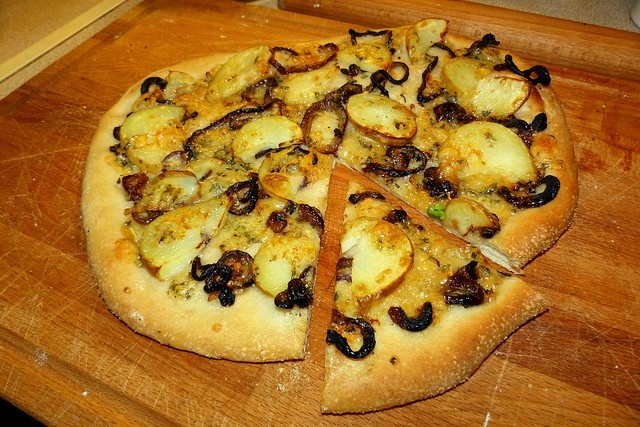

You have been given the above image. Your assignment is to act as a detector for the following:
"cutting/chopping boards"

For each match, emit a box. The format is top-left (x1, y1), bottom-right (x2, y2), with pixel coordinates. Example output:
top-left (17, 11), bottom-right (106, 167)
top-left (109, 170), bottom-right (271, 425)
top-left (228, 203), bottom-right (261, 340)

top-left (1, 0), bottom-right (637, 426)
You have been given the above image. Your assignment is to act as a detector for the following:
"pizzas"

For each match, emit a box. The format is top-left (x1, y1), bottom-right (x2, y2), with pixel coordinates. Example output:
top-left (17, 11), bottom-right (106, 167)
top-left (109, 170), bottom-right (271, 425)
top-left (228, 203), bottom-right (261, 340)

top-left (319, 181), bottom-right (549, 412)
top-left (82, 16), bottom-right (578, 364)
